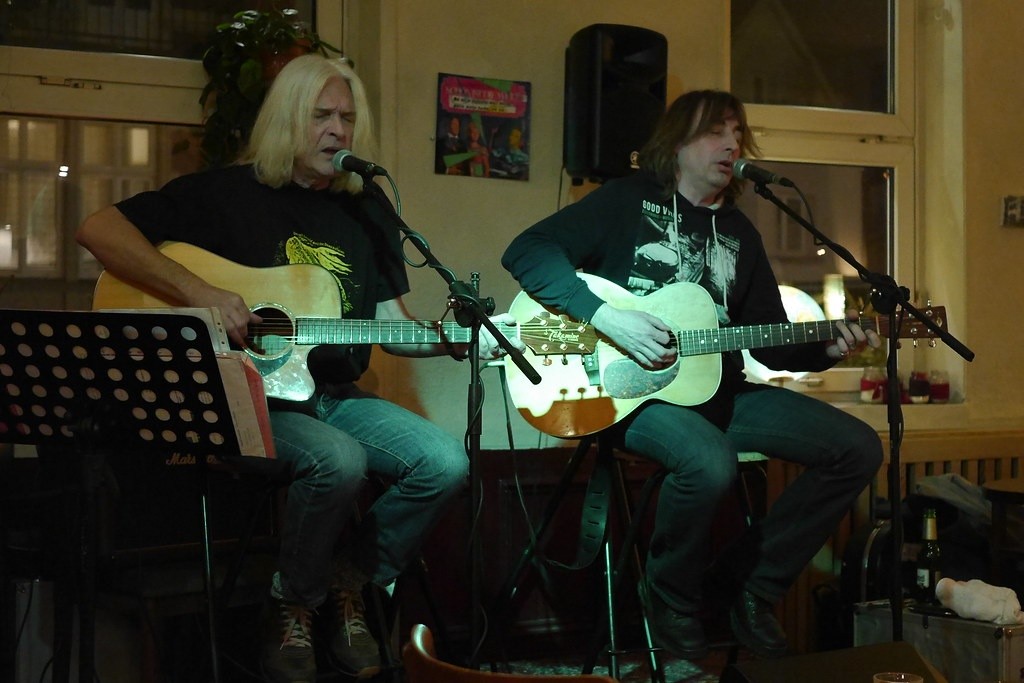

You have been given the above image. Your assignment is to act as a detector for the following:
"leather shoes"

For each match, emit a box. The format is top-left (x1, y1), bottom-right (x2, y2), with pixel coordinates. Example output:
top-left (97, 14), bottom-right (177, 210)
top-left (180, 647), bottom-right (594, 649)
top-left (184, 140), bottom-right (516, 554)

top-left (637, 574), bottom-right (708, 659)
top-left (727, 589), bottom-right (787, 658)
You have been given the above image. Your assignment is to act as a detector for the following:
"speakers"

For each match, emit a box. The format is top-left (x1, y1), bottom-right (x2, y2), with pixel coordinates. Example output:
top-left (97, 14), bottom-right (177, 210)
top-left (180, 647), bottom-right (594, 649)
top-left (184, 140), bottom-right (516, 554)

top-left (562, 23), bottom-right (668, 186)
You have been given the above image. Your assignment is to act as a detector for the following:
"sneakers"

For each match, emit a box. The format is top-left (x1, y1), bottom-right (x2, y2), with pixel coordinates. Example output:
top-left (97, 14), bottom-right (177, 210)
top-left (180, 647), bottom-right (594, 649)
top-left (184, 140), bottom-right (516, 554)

top-left (263, 571), bottom-right (381, 683)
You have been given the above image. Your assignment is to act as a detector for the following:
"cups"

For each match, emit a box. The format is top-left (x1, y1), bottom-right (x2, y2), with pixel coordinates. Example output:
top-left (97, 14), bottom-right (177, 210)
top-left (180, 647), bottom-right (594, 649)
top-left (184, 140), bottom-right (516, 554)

top-left (873, 673), bottom-right (924, 683)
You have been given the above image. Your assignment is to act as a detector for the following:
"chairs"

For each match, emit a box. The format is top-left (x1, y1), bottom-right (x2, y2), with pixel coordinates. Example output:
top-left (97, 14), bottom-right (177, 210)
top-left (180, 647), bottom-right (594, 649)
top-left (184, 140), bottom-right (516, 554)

top-left (189, 459), bottom-right (412, 683)
top-left (498, 443), bottom-right (775, 683)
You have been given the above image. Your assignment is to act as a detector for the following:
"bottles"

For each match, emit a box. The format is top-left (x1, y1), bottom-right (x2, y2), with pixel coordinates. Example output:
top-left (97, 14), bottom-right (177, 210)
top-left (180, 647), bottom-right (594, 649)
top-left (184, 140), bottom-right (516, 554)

top-left (860, 366), bottom-right (905, 403)
top-left (909, 372), bottom-right (930, 403)
top-left (930, 371), bottom-right (950, 403)
top-left (917, 509), bottom-right (943, 608)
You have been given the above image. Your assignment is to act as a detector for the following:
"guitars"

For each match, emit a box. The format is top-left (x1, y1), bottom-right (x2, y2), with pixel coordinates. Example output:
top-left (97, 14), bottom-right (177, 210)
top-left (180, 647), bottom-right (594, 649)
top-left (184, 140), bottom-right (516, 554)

top-left (91, 240), bottom-right (595, 402)
top-left (502, 271), bottom-right (949, 437)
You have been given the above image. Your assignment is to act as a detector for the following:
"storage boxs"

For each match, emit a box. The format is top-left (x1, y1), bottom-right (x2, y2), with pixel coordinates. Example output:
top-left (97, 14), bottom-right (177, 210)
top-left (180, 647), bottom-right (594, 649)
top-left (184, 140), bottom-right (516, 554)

top-left (853, 596), bottom-right (1024, 683)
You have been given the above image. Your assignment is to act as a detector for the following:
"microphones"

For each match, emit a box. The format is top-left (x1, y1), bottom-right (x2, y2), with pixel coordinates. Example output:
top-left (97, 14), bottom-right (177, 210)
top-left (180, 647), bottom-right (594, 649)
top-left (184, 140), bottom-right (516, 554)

top-left (332, 149), bottom-right (388, 177)
top-left (732, 158), bottom-right (793, 188)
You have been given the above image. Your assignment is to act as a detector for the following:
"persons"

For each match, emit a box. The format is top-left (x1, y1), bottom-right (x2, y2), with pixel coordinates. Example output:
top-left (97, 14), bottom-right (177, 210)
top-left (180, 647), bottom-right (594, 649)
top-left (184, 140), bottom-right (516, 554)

top-left (438, 113), bottom-right (530, 182)
top-left (73, 53), bottom-right (526, 683)
top-left (500, 89), bottom-right (883, 661)
